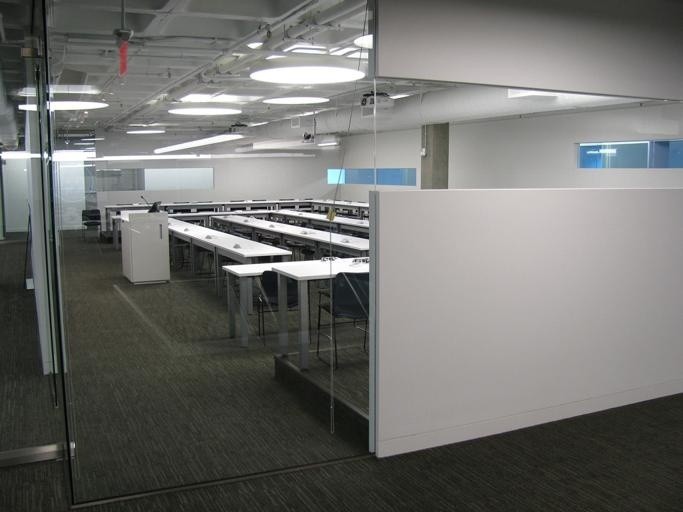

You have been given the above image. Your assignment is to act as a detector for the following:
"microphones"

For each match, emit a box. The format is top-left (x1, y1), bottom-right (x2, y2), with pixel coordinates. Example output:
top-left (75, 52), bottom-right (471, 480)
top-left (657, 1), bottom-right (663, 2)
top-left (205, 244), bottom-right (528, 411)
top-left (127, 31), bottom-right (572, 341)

top-left (141, 196), bottom-right (151, 209)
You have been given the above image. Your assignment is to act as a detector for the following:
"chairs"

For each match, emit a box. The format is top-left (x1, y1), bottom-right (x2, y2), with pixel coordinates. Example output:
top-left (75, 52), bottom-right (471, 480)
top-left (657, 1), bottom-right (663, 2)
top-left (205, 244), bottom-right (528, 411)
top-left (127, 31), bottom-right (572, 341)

top-left (82, 209), bottom-right (102, 241)
top-left (254, 269), bottom-right (310, 338)
top-left (313, 271), bottom-right (369, 370)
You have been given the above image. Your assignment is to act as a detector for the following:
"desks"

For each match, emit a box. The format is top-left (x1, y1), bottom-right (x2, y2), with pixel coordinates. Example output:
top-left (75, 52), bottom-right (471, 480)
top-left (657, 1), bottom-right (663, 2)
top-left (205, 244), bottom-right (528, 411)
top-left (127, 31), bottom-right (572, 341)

top-left (271, 256), bottom-right (369, 370)
top-left (222, 256), bottom-right (339, 348)
top-left (106, 200), bottom-right (369, 295)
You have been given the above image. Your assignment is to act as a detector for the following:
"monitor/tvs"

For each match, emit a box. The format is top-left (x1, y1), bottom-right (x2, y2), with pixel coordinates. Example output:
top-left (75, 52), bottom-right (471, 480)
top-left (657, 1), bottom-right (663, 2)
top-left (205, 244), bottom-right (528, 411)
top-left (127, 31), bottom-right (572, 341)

top-left (148, 201), bottom-right (161, 213)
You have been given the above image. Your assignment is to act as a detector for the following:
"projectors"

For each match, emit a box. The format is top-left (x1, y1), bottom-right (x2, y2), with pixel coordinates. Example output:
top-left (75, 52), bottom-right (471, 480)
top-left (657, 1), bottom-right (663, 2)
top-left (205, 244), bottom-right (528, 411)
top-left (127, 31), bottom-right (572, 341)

top-left (360, 95), bottom-right (394, 109)
top-left (228, 125), bottom-right (249, 133)
